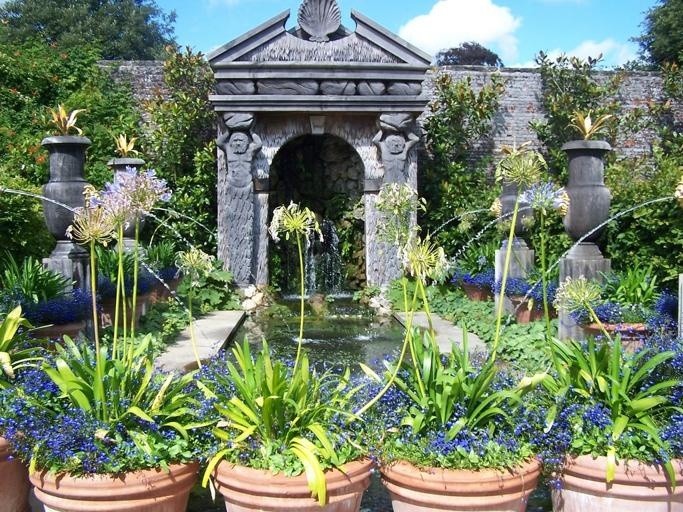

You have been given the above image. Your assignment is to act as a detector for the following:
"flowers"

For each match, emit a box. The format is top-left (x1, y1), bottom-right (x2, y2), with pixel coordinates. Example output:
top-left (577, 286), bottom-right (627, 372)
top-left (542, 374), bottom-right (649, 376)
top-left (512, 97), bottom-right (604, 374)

top-left (0, 104), bottom-right (682, 504)
